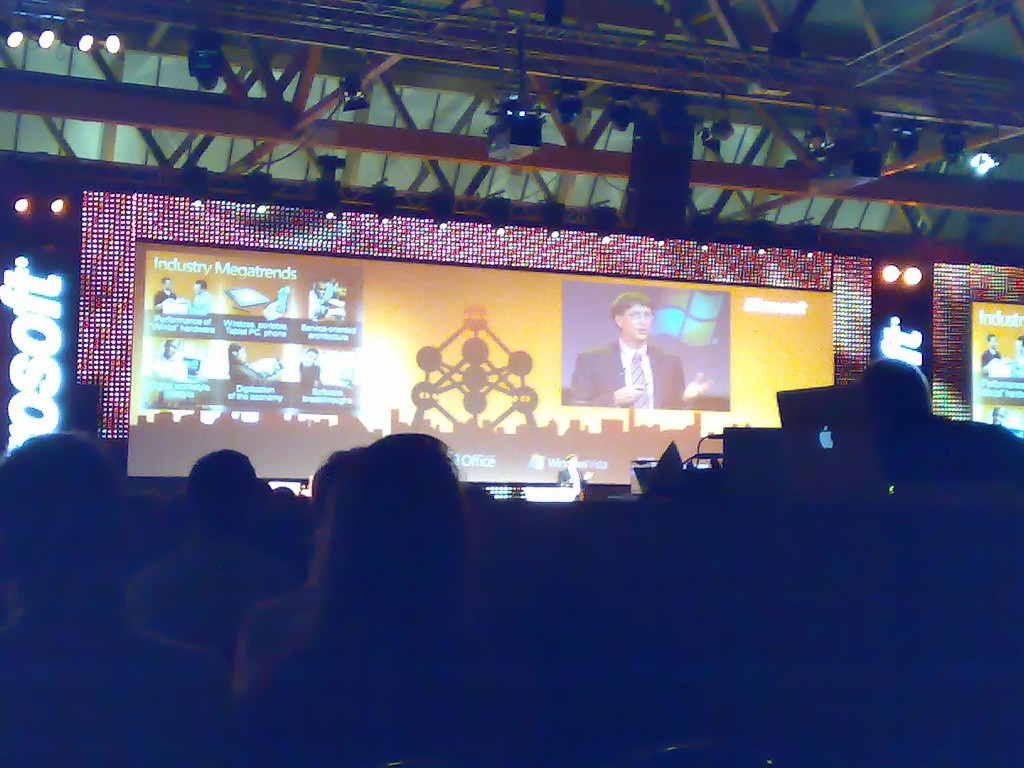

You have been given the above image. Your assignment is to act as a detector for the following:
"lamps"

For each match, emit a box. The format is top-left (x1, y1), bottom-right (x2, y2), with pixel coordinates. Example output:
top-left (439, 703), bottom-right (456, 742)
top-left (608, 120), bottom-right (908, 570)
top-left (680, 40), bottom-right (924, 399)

top-left (343, 77), bottom-right (370, 111)
top-left (95, 33), bottom-right (121, 54)
top-left (60, 31), bottom-right (94, 52)
top-left (186, 29), bottom-right (228, 90)
top-left (24, 22), bottom-right (55, 48)
top-left (0, 22), bottom-right (24, 48)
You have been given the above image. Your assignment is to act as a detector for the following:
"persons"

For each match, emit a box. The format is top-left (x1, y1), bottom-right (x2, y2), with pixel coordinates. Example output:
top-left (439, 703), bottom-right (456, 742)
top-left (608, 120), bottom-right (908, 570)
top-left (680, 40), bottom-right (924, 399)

top-left (151, 278), bottom-right (347, 387)
top-left (981, 335), bottom-right (1001, 374)
top-left (564, 290), bottom-right (714, 409)
top-left (0, 361), bottom-right (1024, 768)
top-left (1016, 336), bottom-right (1024, 370)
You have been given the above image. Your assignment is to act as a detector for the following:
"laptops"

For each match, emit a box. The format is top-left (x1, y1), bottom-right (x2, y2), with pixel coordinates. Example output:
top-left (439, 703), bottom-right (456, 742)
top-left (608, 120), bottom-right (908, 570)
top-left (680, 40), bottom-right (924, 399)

top-left (777, 385), bottom-right (893, 505)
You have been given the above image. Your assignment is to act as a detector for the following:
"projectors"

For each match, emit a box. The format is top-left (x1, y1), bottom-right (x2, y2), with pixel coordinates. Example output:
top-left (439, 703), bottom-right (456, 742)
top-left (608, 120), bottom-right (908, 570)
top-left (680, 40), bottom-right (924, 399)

top-left (488, 117), bottom-right (542, 162)
